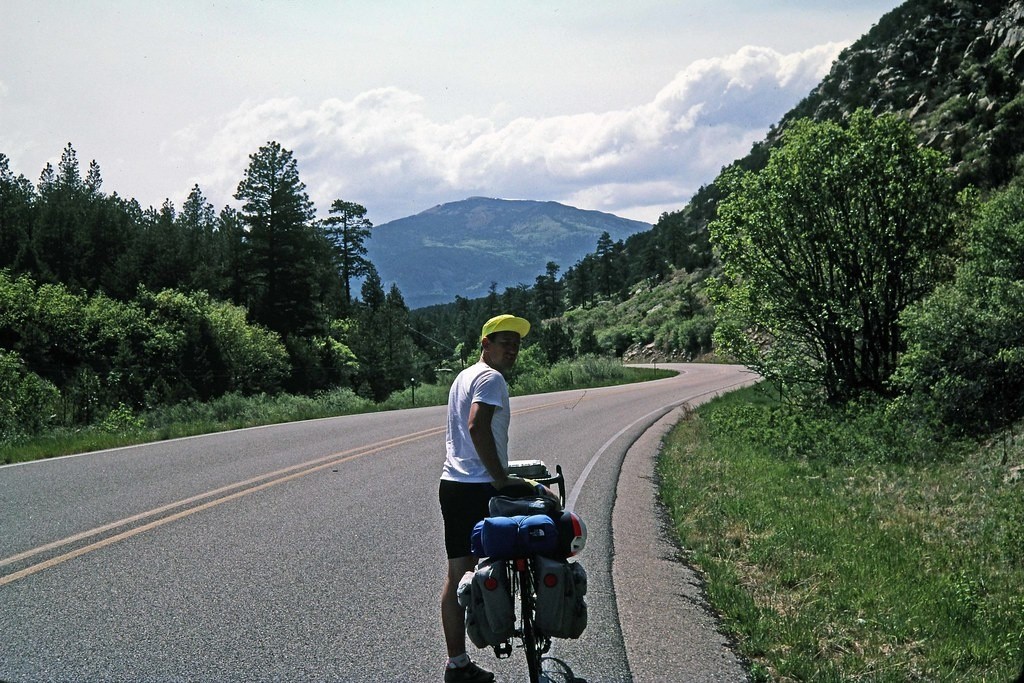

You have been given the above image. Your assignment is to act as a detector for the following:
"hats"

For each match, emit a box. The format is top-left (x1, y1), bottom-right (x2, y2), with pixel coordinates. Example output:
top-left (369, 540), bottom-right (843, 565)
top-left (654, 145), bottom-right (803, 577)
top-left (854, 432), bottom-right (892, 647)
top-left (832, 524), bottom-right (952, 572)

top-left (478, 313), bottom-right (531, 343)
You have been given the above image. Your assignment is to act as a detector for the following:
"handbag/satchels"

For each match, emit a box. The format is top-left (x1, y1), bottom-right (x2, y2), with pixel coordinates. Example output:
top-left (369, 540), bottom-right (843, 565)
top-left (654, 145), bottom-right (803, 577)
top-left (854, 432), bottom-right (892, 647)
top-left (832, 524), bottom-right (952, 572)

top-left (457, 556), bottom-right (515, 649)
top-left (535, 557), bottom-right (588, 639)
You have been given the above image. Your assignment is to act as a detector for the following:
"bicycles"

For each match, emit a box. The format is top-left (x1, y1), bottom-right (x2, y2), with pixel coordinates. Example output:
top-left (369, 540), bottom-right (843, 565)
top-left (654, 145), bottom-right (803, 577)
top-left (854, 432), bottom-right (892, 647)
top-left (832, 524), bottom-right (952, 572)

top-left (488, 464), bottom-right (566, 683)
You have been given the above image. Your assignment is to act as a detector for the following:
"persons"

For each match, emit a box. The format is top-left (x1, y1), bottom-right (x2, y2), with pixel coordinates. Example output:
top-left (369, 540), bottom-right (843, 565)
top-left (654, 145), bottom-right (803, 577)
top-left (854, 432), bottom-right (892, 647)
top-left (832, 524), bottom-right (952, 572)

top-left (439, 314), bottom-right (561, 683)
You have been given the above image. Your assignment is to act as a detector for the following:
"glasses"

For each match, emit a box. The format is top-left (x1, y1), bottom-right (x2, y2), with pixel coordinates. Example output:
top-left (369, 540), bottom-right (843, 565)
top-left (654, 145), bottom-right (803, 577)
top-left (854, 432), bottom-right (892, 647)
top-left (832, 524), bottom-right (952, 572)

top-left (489, 340), bottom-right (523, 347)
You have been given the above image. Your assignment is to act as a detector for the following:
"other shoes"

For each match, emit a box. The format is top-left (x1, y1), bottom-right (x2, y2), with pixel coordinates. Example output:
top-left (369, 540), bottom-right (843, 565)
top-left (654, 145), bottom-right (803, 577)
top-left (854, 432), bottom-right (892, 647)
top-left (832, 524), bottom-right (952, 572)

top-left (444, 656), bottom-right (494, 683)
